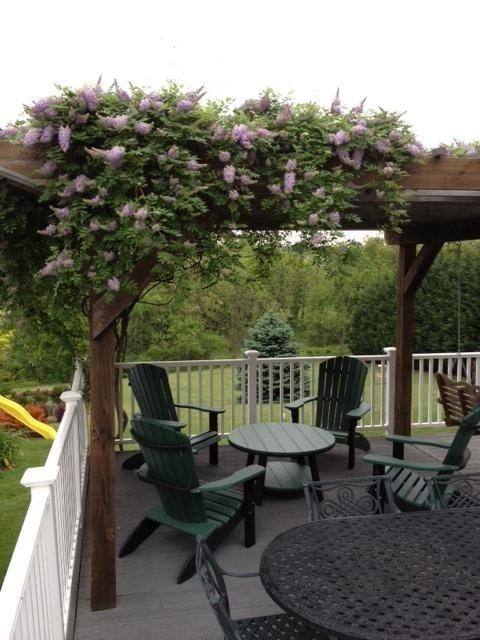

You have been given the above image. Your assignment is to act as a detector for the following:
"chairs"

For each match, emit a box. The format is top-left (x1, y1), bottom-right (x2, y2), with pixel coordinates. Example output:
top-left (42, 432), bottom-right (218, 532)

top-left (193, 532), bottom-right (325, 639)
top-left (434, 369), bottom-right (480, 435)
top-left (300, 475), bottom-right (391, 522)
top-left (122, 362), bottom-right (226, 471)
top-left (283, 356), bottom-right (370, 472)
top-left (119, 417), bottom-right (267, 588)
top-left (362, 405), bottom-right (480, 512)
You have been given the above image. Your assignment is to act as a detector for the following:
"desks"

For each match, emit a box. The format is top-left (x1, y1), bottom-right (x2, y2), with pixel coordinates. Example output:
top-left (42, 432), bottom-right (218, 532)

top-left (228, 423), bottom-right (337, 504)
top-left (258, 510), bottom-right (480, 639)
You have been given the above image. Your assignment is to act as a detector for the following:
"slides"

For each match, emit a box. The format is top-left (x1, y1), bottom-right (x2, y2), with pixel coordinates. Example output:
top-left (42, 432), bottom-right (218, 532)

top-left (0, 395), bottom-right (57, 439)
